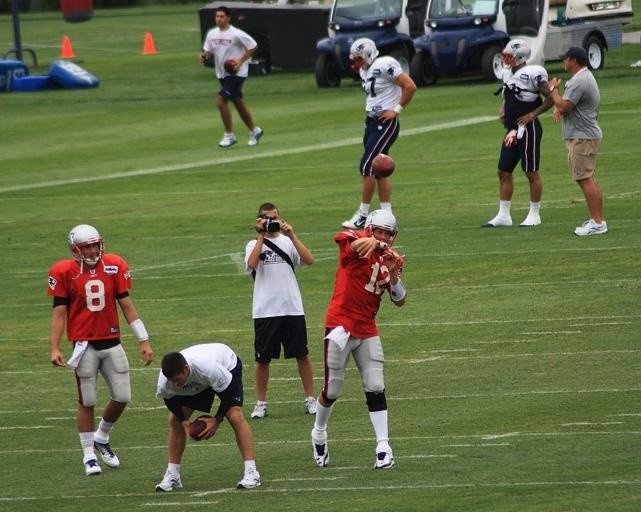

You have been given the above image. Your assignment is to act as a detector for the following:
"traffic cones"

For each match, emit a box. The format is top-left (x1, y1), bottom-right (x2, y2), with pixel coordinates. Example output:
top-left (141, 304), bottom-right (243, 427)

top-left (60, 36), bottom-right (78, 58)
top-left (143, 32), bottom-right (159, 54)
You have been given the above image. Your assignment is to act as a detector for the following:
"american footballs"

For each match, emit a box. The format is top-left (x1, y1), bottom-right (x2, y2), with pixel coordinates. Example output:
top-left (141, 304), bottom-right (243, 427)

top-left (371, 154), bottom-right (396, 178)
top-left (224, 59), bottom-right (238, 73)
top-left (189, 415), bottom-right (212, 441)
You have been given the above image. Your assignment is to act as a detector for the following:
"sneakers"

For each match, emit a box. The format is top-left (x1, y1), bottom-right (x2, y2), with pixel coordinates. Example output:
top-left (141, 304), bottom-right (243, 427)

top-left (246, 127), bottom-right (263, 147)
top-left (304, 395), bottom-right (318, 415)
top-left (235, 468), bottom-right (262, 490)
top-left (95, 431), bottom-right (121, 468)
top-left (371, 444), bottom-right (395, 470)
top-left (311, 429), bottom-right (331, 468)
top-left (83, 456), bottom-right (102, 477)
top-left (217, 131), bottom-right (238, 147)
top-left (250, 401), bottom-right (269, 418)
top-left (573, 220), bottom-right (609, 237)
top-left (155, 469), bottom-right (183, 492)
top-left (519, 216), bottom-right (541, 227)
top-left (480, 214), bottom-right (513, 227)
top-left (340, 211), bottom-right (368, 232)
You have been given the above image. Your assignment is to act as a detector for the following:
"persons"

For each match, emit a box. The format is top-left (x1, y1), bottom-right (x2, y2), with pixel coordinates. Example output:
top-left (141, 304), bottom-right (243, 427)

top-left (46, 224), bottom-right (154, 476)
top-left (155, 342), bottom-right (260, 493)
top-left (199, 6), bottom-right (264, 147)
top-left (548, 47), bottom-right (609, 237)
top-left (341, 38), bottom-right (417, 231)
top-left (244, 203), bottom-right (317, 420)
top-left (311, 209), bottom-right (406, 470)
top-left (482, 38), bottom-right (554, 228)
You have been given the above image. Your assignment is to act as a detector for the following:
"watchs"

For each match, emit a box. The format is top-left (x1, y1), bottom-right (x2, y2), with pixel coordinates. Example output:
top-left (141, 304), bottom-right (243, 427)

top-left (548, 86), bottom-right (558, 93)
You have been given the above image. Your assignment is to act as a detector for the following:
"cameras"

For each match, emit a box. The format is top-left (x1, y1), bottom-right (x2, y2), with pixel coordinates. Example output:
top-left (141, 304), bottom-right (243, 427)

top-left (255, 214), bottom-right (281, 233)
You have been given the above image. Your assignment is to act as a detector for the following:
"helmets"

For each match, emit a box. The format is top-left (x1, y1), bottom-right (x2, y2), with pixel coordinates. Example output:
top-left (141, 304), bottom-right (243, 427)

top-left (67, 223), bottom-right (104, 267)
top-left (365, 209), bottom-right (399, 250)
top-left (348, 37), bottom-right (380, 69)
top-left (500, 38), bottom-right (532, 68)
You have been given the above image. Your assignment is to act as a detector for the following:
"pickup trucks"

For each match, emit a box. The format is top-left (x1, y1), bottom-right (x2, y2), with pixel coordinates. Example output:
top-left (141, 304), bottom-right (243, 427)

top-left (314, 1), bottom-right (412, 89)
top-left (410, 0), bottom-right (633, 88)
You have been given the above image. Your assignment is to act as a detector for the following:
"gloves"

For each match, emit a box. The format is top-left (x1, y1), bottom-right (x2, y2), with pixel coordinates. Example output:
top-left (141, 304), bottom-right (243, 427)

top-left (503, 127), bottom-right (518, 148)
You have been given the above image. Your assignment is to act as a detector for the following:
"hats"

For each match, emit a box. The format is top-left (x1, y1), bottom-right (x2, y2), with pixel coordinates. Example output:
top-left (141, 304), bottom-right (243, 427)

top-left (559, 46), bottom-right (588, 64)
